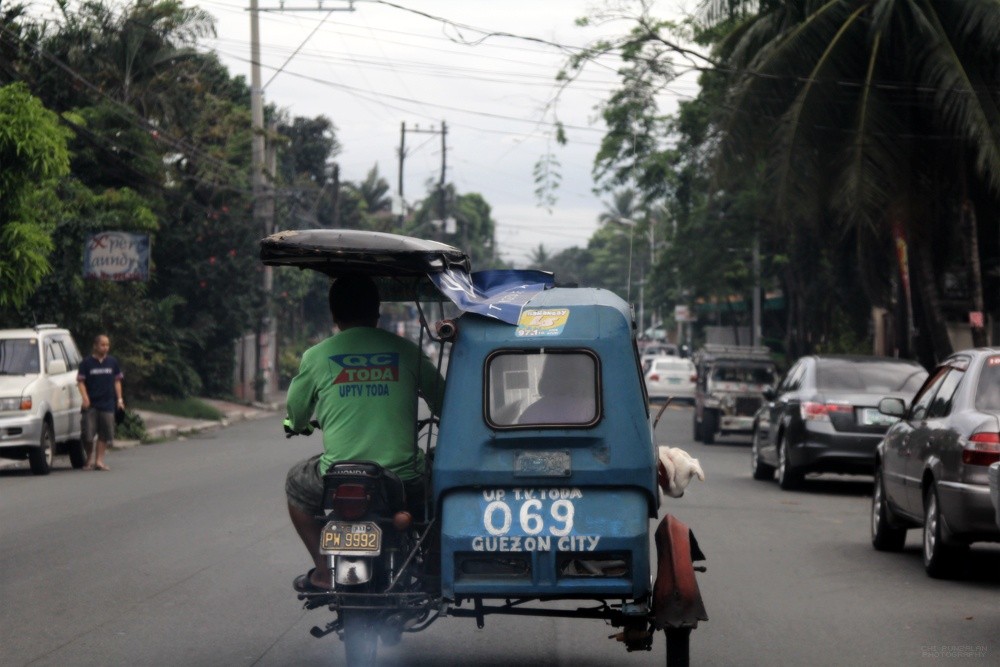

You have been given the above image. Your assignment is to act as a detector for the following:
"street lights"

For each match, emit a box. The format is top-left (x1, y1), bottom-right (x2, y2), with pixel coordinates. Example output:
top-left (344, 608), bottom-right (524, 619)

top-left (613, 197), bottom-right (678, 328)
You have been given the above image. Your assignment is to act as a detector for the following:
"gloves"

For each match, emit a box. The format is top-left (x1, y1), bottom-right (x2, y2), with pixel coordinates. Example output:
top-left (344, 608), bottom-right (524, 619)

top-left (284, 416), bottom-right (314, 436)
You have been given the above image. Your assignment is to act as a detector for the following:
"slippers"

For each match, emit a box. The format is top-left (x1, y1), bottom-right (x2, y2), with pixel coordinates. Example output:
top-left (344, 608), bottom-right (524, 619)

top-left (293, 568), bottom-right (333, 594)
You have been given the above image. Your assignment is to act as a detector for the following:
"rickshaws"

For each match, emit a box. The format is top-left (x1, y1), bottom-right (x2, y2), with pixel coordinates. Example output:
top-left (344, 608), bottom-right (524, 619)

top-left (260, 228), bottom-right (710, 667)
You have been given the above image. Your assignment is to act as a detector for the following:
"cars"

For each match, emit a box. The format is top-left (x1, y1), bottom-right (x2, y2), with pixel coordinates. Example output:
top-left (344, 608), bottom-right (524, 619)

top-left (871, 345), bottom-right (1000, 579)
top-left (752, 353), bottom-right (931, 490)
top-left (634, 320), bottom-right (699, 406)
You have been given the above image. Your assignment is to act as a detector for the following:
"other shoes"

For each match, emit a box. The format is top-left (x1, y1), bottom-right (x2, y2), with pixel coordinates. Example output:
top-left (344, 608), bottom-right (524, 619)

top-left (83, 464), bottom-right (96, 471)
top-left (97, 464), bottom-right (110, 470)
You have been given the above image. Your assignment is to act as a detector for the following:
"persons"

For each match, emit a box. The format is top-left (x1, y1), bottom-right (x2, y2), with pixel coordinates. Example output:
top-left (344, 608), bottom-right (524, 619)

top-left (282, 272), bottom-right (447, 594)
top-left (516, 353), bottom-right (596, 424)
top-left (77, 334), bottom-right (126, 471)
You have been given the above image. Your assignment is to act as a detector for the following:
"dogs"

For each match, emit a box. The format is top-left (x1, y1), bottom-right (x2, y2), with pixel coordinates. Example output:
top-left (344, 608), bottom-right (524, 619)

top-left (658, 445), bottom-right (705, 498)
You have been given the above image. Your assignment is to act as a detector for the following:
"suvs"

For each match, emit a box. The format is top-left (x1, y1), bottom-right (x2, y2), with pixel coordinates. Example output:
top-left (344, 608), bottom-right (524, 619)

top-left (692, 342), bottom-right (784, 444)
top-left (0, 324), bottom-right (94, 475)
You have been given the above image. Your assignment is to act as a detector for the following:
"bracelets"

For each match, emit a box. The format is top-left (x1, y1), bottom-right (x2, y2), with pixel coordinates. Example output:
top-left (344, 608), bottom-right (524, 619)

top-left (118, 399), bottom-right (123, 403)
